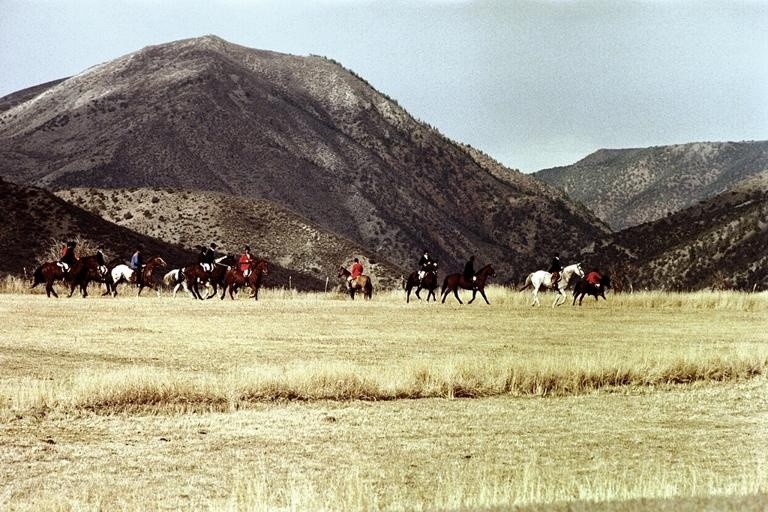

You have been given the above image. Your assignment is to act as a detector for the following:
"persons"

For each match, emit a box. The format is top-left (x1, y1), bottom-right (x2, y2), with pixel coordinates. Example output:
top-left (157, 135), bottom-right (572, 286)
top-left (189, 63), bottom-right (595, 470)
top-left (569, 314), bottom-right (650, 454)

top-left (345, 258), bottom-right (363, 294)
top-left (548, 253), bottom-right (563, 290)
top-left (418, 250), bottom-right (432, 288)
top-left (59, 241), bottom-right (109, 282)
top-left (239, 246), bottom-right (255, 287)
top-left (198, 246), bottom-right (207, 284)
top-left (464, 256), bottom-right (479, 289)
top-left (585, 269), bottom-right (603, 290)
top-left (130, 243), bottom-right (146, 287)
top-left (203, 243), bottom-right (217, 282)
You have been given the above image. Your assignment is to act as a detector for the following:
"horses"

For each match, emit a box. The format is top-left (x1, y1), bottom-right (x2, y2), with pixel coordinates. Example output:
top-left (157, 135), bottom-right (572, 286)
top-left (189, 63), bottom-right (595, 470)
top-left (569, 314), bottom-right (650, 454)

top-left (405, 259), bottom-right (440, 303)
top-left (524, 262), bottom-right (585, 309)
top-left (29, 252), bottom-right (269, 300)
top-left (573, 272), bottom-right (612, 305)
top-left (338, 266), bottom-right (372, 301)
top-left (441, 264), bottom-right (496, 304)
